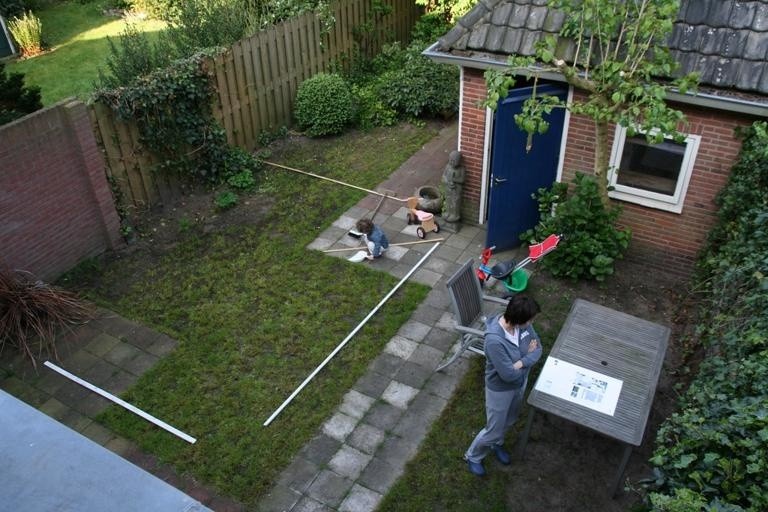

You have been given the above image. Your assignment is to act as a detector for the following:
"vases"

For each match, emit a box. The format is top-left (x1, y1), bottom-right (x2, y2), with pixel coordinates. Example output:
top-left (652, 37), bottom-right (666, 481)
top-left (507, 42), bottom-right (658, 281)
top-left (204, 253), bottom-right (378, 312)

top-left (414, 185), bottom-right (442, 213)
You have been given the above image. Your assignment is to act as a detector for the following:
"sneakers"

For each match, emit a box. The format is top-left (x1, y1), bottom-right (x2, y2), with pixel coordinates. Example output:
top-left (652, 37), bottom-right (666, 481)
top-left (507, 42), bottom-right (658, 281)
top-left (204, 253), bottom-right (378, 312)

top-left (493, 449), bottom-right (511, 465)
top-left (469, 460), bottom-right (484, 477)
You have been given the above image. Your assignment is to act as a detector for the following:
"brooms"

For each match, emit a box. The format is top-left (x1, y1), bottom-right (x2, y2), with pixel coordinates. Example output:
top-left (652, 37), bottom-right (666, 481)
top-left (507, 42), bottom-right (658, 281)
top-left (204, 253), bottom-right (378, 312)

top-left (370, 185), bottom-right (397, 224)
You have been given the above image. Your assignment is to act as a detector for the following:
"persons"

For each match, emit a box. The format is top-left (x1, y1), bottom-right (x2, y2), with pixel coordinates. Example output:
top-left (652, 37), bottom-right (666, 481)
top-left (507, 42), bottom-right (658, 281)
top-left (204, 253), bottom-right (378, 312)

top-left (357, 218), bottom-right (388, 261)
top-left (463, 292), bottom-right (542, 476)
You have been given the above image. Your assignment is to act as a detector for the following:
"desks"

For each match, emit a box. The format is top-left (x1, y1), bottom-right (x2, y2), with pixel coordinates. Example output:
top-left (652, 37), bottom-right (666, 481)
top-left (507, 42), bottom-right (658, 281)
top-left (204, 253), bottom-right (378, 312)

top-left (515, 299), bottom-right (673, 501)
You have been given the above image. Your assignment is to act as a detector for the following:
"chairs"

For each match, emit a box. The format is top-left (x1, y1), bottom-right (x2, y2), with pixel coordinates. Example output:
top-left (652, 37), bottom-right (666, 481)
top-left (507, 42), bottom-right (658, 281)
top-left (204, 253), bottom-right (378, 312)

top-left (435, 257), bottom-right (510, 373)
